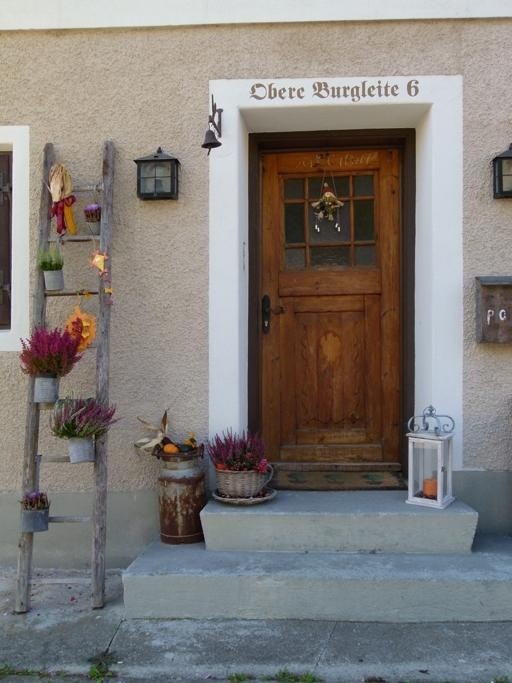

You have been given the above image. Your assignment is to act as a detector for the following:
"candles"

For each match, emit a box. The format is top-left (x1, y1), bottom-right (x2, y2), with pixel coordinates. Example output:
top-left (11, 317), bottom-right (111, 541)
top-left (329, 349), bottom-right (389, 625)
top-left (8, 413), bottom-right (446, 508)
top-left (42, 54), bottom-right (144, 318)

top-left (423, 478), bottom-right (436, 497)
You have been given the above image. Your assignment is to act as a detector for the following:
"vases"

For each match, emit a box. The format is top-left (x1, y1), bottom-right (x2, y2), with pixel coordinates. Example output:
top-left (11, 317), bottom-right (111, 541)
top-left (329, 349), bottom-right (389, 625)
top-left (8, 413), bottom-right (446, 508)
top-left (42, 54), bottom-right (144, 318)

top-left (66, 437), bottom-right (96, 463)
top-left (213, 465), bottom-right (274, 498)
top-left (157, 442), bottom-right (207, 545)
top-left (33, 376), bottom-right (59, 403)
top-left (88, 221), bottom-right (100, 233)
top-left (19, 509), bottom-right (48, 533)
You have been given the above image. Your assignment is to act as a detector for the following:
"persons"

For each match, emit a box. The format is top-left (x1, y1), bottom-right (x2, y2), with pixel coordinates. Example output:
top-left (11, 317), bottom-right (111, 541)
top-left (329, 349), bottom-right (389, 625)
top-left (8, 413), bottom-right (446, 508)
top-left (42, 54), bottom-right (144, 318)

top-left (313, 180), bottom-right (345, 233)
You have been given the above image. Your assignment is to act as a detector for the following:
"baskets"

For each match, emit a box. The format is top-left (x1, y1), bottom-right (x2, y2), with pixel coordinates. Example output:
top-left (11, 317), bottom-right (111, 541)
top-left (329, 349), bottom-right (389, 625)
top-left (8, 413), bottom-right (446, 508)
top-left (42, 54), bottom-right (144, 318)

top-left (212, 463), bottom-right (277, 505)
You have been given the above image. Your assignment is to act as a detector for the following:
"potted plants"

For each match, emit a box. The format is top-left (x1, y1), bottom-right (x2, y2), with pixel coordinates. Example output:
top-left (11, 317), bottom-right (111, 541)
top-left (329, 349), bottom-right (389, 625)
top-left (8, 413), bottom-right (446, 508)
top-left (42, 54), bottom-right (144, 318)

top-left (37, 246), bottom-right (65, 290)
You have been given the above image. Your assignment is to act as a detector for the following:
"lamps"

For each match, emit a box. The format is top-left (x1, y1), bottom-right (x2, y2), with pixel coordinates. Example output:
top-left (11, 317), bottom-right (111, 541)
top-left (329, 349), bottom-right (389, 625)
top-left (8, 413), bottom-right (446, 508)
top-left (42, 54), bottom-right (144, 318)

top-left (494, 142), bottom-right (512, 198)
top-left (134, 146), bottom-right (181, 200)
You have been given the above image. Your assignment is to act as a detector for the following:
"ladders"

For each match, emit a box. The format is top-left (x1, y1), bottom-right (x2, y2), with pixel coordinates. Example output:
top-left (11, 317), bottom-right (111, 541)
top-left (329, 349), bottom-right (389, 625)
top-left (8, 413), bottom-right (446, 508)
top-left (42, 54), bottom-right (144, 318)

top-left (15, 139), bottom-right (117, 615)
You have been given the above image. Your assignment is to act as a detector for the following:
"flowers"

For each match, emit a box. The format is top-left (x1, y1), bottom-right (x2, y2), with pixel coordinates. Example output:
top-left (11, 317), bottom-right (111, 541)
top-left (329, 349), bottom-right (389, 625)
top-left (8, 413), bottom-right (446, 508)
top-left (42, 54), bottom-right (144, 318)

top-left (84, 202), bottom-right (101, 221)
top-left (206, 426), bottom-right (269, 475)
top-left (134, 410), bottom-right (197, 460)
top-left (16, 490), bottom-right (50, 509)
top-left (50, 395), bottom-right (122, 436)
top-left (18, 318), bottom-right (83, 377)
